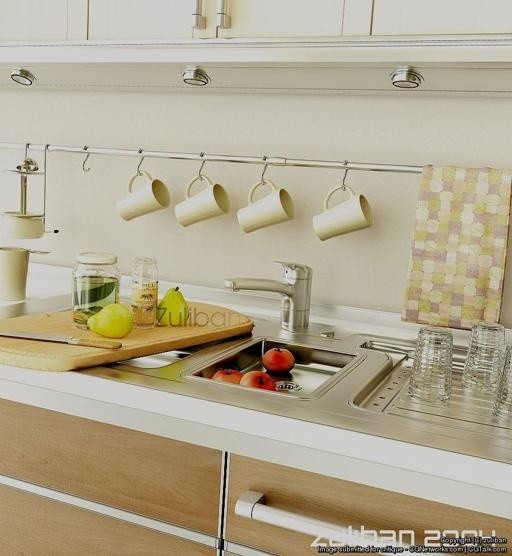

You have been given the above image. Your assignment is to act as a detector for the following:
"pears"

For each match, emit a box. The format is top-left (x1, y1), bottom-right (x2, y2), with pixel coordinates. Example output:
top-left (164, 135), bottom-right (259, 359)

top-left (86, 302), bottom-right (133, 338)
top-left (157, 287), bottom-right (189, 326)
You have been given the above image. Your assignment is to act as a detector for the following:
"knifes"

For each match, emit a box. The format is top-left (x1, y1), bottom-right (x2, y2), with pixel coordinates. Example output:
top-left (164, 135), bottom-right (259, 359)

top-left (0, 331), bottom-right (123, 349)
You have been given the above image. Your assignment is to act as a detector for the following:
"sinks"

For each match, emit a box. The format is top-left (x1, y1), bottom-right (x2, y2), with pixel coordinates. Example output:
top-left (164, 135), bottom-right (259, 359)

top-left (180, 335), bottom-right (367, 399)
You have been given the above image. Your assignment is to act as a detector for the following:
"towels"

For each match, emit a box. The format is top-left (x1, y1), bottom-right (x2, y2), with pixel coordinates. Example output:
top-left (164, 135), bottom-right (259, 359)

top-left (399, 162), bottom-right (512, 331)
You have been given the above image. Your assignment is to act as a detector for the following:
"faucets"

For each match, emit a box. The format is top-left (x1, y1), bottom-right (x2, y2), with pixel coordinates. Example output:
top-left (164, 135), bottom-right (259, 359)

top-left (224, 260), bottom-right (313, 333)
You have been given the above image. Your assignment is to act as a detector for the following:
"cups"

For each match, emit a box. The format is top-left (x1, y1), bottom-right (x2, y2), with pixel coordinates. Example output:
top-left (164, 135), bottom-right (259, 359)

top-left (118, 170), bottom-right (172, 221)
top-left (311, 184), bottom-right (374, 241)
top-left (0, 247), bottom-right (30, 300)
top-left (236, 179), bottom-right (295, 233)
top-left (175, 175), bottom-right (231, 227)
top-left (409, 319), bottom-right (512, 427)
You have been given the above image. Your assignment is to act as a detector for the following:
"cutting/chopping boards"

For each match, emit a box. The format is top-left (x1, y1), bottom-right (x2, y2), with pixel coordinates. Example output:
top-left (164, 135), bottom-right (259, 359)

top-left (1, 302), bottom-right (255, 373)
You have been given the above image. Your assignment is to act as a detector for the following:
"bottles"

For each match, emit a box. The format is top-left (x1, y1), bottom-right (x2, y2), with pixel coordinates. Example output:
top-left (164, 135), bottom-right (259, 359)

top-left (129, 256), bottom-right (158, 330)
top-left (72, 252), bottom-right (122, 330)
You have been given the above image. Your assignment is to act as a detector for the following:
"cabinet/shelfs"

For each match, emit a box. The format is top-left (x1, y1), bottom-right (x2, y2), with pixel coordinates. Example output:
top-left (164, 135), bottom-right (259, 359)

top-left (0, 0), bottom-right (512, 41)
top-left (1, 396), bottom-right (227, 556)
top-left (220, 451), bottom-right (511, 555)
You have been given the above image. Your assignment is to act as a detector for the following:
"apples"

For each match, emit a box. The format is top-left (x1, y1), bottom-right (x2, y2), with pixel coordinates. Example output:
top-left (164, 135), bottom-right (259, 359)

top-left (212, 369), bottom-right (242, 384)
top-left (262, 348), bottom-right (295, 373)
top-left (240, 371), bottom-right (276, 391)
